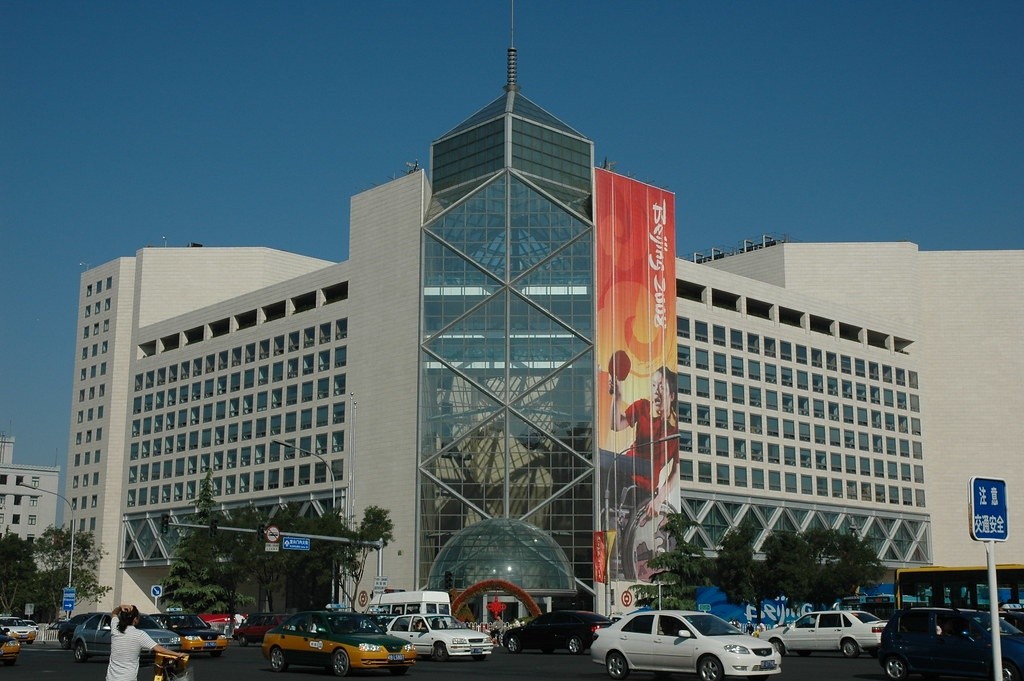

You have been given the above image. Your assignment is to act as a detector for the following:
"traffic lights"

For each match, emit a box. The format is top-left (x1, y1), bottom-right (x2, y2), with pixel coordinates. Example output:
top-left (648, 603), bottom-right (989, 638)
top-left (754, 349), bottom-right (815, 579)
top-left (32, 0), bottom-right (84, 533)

top-left (209, 518), bottom-right (217, 535)
top-left (161, 513), bottom-right (169, 535)
top-left (257, 522), bottom-right (265, 541)
top-left (444, 571), bottom-right (453, 590)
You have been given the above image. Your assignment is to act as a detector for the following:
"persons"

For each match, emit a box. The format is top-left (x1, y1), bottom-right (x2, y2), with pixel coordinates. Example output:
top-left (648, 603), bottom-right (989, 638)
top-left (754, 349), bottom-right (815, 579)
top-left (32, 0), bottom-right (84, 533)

top-left (600, 367), bottom-right (680, 517)
top-left (728, 617), bottom-right (778, 638)
top-left (297, 619), bottom-right (305, 632)
top-left (105, 604), bottom-right (184, 681)
top-left (416, 620), bottom-right (423, 630)
top-left (514, 618), bottom-right (520, 627)
top-left (490, 616), bottom-right (503, 648)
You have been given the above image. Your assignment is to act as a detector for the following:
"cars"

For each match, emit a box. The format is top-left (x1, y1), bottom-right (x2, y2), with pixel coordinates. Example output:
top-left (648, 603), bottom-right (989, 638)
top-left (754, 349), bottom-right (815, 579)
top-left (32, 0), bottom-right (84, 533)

top-left (232, 611), bottom-right (416, 677)
top-left (0, 626), bottom-right (20, 663)
top-left (591, 609), bottom-right (781, 681)
top-left (999, 610), bottom-right (1024, 632)
top-left (385, 613), bottom-right (494, 661)
top-left (47, 612), bottom-right (227, 663)
top-left (503, 610), bottom-right (614, 655)
top-left (0, 616), bottom-right (40, 645)
top-left (759, 610), bottom-right (888, 658)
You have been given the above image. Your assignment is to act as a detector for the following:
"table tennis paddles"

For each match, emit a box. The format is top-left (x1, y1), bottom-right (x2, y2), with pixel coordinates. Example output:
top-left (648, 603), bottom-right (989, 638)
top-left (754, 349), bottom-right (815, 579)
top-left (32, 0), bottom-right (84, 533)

top-left (608, 350), bottom-right (631, 394)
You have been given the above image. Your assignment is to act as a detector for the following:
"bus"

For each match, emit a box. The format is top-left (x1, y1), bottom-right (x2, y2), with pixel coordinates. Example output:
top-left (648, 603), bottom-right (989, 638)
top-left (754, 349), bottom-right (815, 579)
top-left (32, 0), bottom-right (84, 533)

top-left (832, 594), bottom-right (918, 616)
top-left (895, 564), bottom-right (1024, 612)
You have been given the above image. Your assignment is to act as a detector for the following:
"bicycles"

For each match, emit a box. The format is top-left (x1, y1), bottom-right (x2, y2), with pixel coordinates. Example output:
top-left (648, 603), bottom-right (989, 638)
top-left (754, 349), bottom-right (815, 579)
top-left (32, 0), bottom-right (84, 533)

top-left (152, 651), bottom-right (187, 681)
top-left (487, 628), bottom-right (504, 648)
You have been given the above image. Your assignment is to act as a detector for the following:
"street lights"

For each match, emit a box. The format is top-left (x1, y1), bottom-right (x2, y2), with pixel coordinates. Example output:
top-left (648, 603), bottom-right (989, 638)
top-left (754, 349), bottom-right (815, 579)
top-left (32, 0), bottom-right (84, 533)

top-left (272, 438), bottom-right (336, 608)
top-left (605, 433), bottom-right (681, 617)
top-left (18, 482), bottom-right (74, 618)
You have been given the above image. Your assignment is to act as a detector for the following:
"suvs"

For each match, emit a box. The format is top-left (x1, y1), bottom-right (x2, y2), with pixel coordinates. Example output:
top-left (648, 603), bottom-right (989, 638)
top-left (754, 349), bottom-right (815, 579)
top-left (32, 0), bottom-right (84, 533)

top-left (880, 608), bottom-right (1024, 681)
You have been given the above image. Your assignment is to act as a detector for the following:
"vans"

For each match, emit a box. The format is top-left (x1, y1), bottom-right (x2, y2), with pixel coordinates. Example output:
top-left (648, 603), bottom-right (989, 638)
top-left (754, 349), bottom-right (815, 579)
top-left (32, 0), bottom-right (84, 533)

top-left (363, 591), bottom-right (451, 626)
